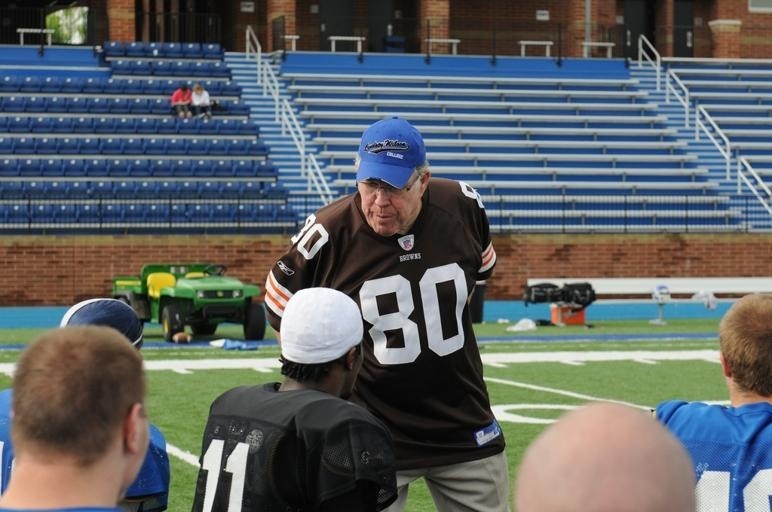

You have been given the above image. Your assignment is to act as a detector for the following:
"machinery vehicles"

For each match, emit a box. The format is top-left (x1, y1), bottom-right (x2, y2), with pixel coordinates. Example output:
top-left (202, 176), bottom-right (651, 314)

top-left (111, 262), bottom-right (266, 343)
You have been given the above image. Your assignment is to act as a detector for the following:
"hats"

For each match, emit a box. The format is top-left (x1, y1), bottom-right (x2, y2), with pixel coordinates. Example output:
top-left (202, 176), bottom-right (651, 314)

top-left (279, 287), bottom-right (365, 365)
top-left (356, 116), bottom-right (426, 190)
top-left (60, 298), bottom-right (144, 348)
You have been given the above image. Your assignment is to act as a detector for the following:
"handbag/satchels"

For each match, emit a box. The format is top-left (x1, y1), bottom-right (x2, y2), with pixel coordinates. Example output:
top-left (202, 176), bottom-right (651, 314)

top-left (528, 283), bottom-right (596, 304)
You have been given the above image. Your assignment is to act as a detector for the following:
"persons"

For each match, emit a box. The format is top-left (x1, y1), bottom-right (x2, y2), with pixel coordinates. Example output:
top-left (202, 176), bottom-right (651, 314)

top-left (515, 400), bottom-right (696, 512)
top-left (264, 116), bottom-right (509, 512)
top-left (191, 287), bottom-right (399, 512)
top-left (0, 298), bottom-right (170, 512)
top-left (650, 293), bottom-right (772, 512)
top-left (171, 84), bottom-right (211, 120)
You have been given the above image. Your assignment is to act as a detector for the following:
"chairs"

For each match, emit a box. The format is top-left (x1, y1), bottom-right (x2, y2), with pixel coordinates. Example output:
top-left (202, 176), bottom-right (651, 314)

top-left (0, 38), bottom-right (299, 233)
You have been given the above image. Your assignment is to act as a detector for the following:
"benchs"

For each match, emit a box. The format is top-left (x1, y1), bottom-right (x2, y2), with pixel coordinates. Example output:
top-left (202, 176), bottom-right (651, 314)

top-left (281, 59), bottom-right (771, 235)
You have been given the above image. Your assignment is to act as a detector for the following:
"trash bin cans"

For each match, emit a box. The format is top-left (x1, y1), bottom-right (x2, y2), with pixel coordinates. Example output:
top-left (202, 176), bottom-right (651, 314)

top-left (384, 35), bottom-right (406, 53)
